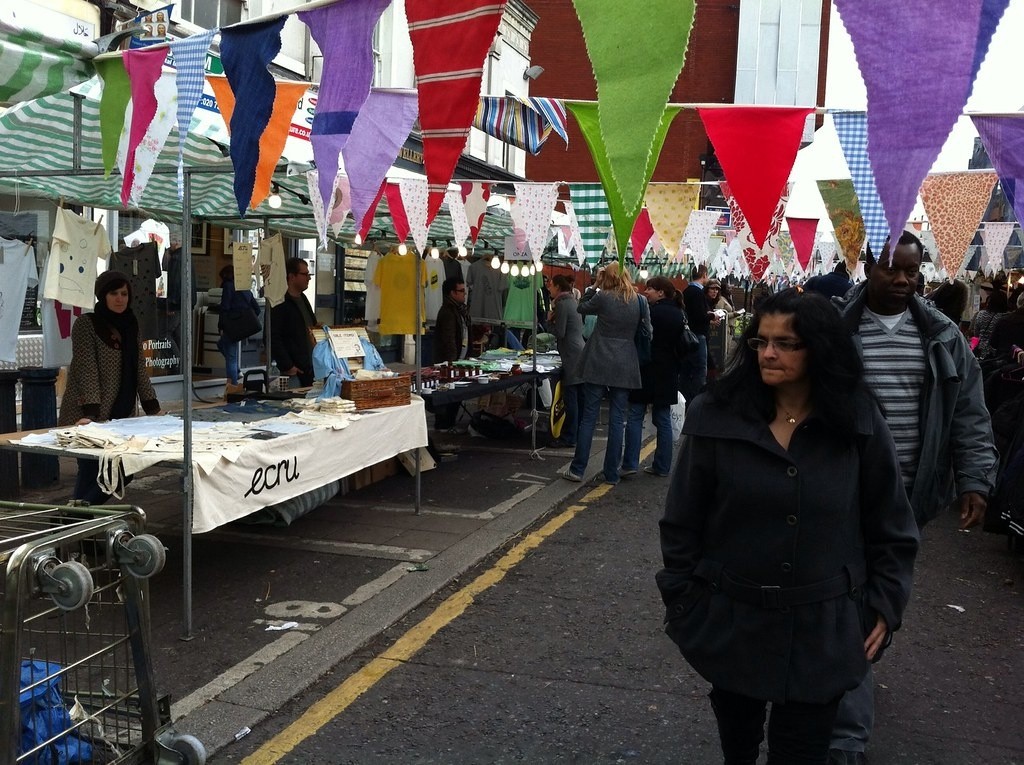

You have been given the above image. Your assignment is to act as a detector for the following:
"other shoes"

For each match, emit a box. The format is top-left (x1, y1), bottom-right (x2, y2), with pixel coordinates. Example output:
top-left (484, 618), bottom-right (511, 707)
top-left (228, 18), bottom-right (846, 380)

top-left (439, 424), bottom-right (465, 433)
top-left (618, 466), bottom-right (638, 476)
top-left (551, 438), bottom-right (576, 448)
top-left (644, 465), bottom-right (668, 476)
top-left (827, 748), bottom-right (867, 765)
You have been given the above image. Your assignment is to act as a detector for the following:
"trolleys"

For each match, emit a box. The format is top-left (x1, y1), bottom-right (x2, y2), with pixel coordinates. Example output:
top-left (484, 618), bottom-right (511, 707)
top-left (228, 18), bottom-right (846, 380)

top-left (0, 497), bottom-right (207, 765)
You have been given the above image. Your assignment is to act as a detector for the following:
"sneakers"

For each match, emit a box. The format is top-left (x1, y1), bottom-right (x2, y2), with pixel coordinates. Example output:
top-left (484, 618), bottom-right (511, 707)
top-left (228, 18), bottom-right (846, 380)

top-left (562, 468), bottom-right (582, 481)
top-left (595, 471), bottom-right (619, 484)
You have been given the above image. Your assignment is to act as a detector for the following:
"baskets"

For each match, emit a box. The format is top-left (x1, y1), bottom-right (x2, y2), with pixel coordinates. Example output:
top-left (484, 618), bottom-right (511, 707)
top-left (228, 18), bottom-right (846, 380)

top-left (339, 373), bottom-right (411, 409)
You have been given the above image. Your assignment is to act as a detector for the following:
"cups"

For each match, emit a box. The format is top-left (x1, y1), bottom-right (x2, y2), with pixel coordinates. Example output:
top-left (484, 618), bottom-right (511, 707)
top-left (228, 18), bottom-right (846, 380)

top-left (478, 378), bottom-right (488, 384)
top-left (420, 389), bottom-right (432, 394)
top-left (438, 387), bottom-right (448, 392)
top-left (499, 374), bottom-right (509, 379)
top-left (445, 383), bottom-right (454, 390)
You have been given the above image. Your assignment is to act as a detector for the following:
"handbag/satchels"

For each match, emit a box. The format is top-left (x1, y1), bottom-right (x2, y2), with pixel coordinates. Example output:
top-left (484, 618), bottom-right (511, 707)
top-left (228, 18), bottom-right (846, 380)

top-left (223, 311), bottom-right (262, 342)
top-left (670, 390), bottom-right (686, 441)
top-left (970, 337), bottom-right (980, 351)
top-left (682, 309), bottom-right (699, 354)
top-left (636, 293), bottom-right (651, 354)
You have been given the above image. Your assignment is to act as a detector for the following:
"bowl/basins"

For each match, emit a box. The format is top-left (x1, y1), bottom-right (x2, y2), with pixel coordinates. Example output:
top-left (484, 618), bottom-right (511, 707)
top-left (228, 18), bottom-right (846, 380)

top-left (453, 382), bottom-right (472, 387)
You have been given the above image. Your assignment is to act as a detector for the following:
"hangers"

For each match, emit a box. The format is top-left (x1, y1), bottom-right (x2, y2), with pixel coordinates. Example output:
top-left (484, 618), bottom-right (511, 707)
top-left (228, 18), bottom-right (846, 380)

top-left (369, 239), bottom-right (495, 262)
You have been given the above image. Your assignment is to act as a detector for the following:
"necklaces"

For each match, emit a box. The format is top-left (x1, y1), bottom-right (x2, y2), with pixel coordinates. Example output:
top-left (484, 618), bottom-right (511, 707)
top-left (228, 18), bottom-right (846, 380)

top-left (779, 404), bottom-right (799, 423)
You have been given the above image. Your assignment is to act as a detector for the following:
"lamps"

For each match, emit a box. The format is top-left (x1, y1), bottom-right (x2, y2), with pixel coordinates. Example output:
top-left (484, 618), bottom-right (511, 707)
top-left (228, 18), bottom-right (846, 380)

top-left (523, 65), bottom-right (545, 80)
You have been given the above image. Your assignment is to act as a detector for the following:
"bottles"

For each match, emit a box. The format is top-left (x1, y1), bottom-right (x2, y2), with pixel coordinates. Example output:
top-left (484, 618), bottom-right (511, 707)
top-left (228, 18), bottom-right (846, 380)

top-left (448, 363), bottom-right (459, 379)
top-left (440, 365), bottom-right (449, 377)
top-left (409, 376), bottom-right (439, 393)
top-left (474, 365), bottom-right (483, 375)
top-left (458, 365), bottom-right (476, 377)
top-left (268, 361), bottom-right (280, 393)
top-left (511, 364), bottom-right (522, 375)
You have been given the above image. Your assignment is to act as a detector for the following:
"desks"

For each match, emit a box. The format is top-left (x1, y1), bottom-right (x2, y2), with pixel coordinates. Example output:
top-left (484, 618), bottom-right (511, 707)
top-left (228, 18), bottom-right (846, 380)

top-left (410, 351), bottom-right (562, 451)
top-left (0, 399), bottom-right (428, 588)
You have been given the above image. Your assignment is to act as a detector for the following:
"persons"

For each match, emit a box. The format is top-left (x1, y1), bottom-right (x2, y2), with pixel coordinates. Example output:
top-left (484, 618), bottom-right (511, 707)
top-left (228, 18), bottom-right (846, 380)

top-left (263, 258), bottom-right (319, 390)
top-left (50, 270), bottom-right (162, 552)
top-left (535, 260), bottom-right (858, 485)
top-left (829, 228), bottom-right (999, 535)
top-left (655, 287), bottom-right (921, 765)
top-left (923, 266), bottom-right (1024, 536)
top-left (217, 267), bottom-right (262, 386)
top-left (433, 277), bottom-right (470, 432)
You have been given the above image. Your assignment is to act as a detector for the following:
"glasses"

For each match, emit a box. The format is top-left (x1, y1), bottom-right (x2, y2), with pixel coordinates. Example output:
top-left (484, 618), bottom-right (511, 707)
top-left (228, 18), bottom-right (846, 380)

top-left (454, 289), bottom-right (466, 293)
top-left (296, 272), bottom-right (309, 277)
top-left (747, 338), bottom-right (806, 353)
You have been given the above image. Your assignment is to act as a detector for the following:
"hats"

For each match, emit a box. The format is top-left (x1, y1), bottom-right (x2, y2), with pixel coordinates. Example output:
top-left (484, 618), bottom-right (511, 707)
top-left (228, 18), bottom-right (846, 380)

top-left (706, 278), bottom-right (722, 288)
top-left (95, 270), bottom-right (130, 296)
